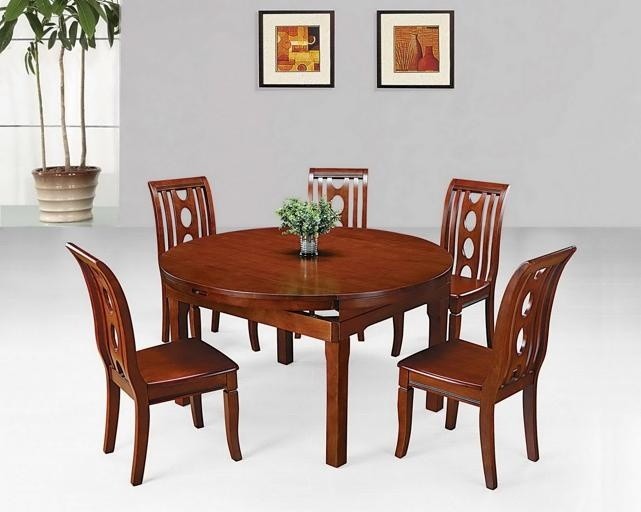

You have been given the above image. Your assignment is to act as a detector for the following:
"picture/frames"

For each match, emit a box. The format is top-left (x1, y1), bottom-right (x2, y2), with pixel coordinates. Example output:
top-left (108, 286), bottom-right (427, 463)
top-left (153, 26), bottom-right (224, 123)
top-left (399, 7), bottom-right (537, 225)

top-left (258, 10), bottom-right (334, 88)
top-left (376, 10), bottom-right (454, 89)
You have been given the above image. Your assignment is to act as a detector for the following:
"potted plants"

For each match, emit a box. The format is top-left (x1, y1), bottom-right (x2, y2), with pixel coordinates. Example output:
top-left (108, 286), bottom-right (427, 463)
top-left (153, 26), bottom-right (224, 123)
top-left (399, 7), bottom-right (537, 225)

top-left (272, 196), bottom-right (344, 257)
top-left (1, 0), bottom-right (120, 223)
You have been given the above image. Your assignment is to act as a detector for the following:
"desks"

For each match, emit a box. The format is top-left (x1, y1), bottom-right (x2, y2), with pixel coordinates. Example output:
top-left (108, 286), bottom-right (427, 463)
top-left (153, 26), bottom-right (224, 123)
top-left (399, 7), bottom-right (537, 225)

top-left (160, 227), bottom-right (455, 467)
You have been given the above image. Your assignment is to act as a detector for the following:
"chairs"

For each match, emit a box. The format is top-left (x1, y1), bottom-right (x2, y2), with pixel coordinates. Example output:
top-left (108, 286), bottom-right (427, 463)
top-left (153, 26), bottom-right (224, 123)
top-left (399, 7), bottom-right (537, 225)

top-left (65, 242), bottom-right (242, 485)
top-left (391, 178), bottom-right (510, 357)
top-left (295, 167), bottom-right (368, 341)
top-left (147, 175), bottom-right (260, 352)
top-left (394, 245), bottom-right (577, 488)
